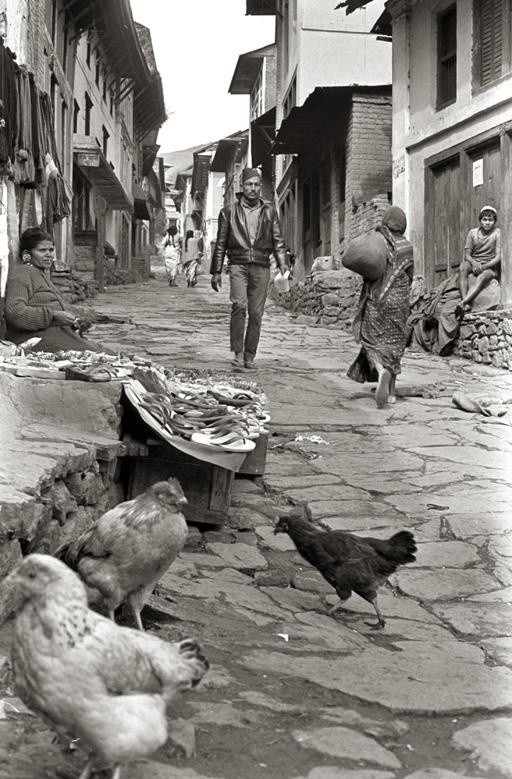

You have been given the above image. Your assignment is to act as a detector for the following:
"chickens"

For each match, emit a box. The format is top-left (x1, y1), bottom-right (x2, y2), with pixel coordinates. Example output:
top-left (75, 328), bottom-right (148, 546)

top-left (51, 474), bottom-right (190, 634)
top-left (1, 551), bottom-right (211, 778)
top-left (271, 514), bottom-right (417, 632)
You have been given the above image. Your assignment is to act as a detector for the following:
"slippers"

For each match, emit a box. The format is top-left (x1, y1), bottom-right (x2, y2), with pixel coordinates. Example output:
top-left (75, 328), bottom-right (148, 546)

top-left (126, 352), bottom-right (270, 452)
top-left (2, 337), bottom-right (134, 381)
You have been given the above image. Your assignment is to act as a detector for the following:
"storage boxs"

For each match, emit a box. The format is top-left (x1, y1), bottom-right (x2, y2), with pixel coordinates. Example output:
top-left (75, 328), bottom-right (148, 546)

top-left (237, 433), bottom-right (269, 475)
top-left (126, 444), bottom-right (234, 526)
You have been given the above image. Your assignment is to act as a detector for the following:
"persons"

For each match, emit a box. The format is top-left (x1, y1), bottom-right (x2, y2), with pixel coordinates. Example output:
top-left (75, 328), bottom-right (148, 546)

top-left (181, 230), bottom-right (199, 287)
top-left (457, 206), bottom-right (501, 312)
top-left (345, 206), bottom-right (414, 408)
top-left (210, 168), bottom-right (289, 369)
top-left (163, 226), bottom-right (181, 286)
top-left (5, 227), bottom-right (118, 356)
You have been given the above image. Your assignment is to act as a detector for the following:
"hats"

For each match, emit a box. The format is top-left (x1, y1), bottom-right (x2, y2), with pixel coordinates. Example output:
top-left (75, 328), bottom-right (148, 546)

top-left (480, 205), bottom-right (496, 214)
top-left (243, 168), bottom-right (259, 183)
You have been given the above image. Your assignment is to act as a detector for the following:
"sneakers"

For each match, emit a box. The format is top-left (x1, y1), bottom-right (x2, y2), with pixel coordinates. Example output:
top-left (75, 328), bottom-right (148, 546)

top-left (374, 368), bottom-right (396, 409)
